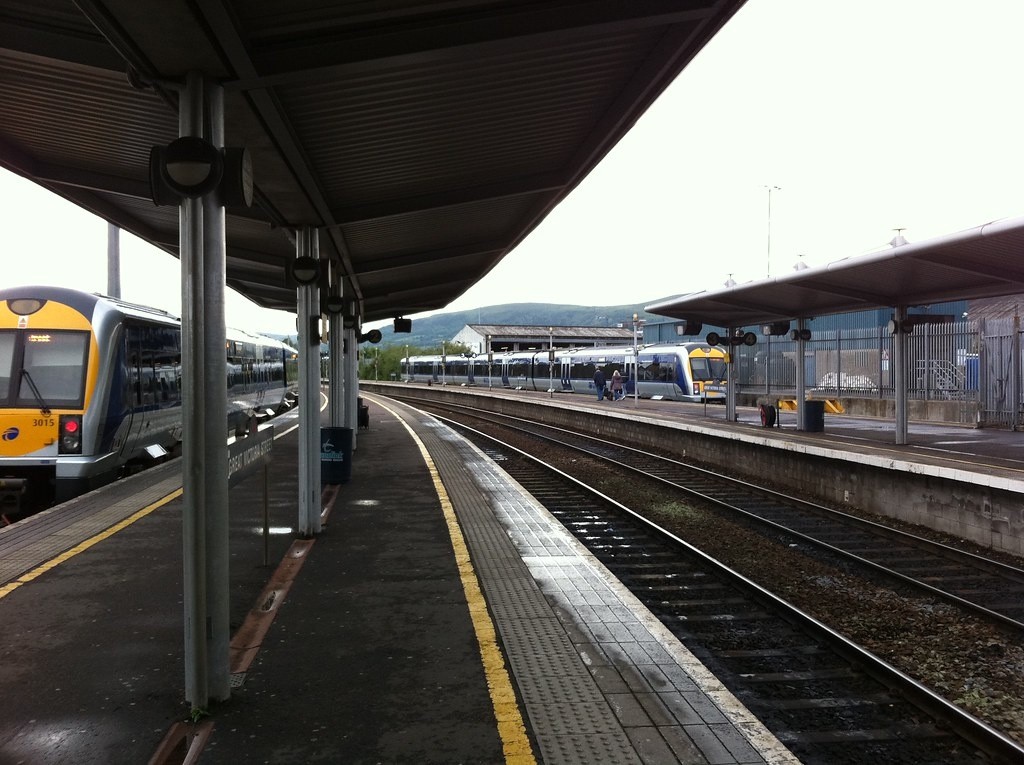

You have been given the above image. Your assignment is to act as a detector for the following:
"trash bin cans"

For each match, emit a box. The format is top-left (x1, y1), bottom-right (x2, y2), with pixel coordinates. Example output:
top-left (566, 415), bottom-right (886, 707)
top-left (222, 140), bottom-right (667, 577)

top-left (320, 426), bottom-right (354, 484)
top-left (428, 379), bottom-right (431, 386)
top-left (357, 396), bottom-right (364, 408)
top-left (804, 400), bottom-right (826, 432)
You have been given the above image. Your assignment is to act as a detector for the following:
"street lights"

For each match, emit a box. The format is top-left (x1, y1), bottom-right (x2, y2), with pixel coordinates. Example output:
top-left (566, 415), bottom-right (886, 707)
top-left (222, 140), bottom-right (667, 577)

top-left (549, 326), bottom-right (553, 398)
top-left (405, 344), bottom-right (409, 385)
top-left (375, 347), bottom-right (378, 383)
top-left (632, 313), bottom-right (639, 409)
top-left (442, 340), bottom-right (445, 387)
top-left (488, 334), bottom-right (492, 391)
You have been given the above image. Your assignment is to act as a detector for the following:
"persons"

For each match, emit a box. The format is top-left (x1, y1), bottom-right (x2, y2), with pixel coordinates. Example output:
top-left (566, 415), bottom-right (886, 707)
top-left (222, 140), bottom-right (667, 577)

top-left (610, 370), bottom-right (623, 402)
top-left (594, 367), bottom-right (607, 401)
top-left (620, 368), bottom-right (629, 400)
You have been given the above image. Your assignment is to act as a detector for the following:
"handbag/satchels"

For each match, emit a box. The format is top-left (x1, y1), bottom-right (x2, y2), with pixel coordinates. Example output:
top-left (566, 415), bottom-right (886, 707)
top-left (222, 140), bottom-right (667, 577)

top-left (607, 390), bottom-right (613, 401)
top-left (619, 389), bottom-right (622, 394)
top-left (603, 387), bottom-right (608, 396)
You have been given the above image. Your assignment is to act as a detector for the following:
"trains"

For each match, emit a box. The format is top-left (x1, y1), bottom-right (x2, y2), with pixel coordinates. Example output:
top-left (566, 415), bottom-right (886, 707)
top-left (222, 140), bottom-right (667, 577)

top-left (0, 283), bottom-right (299, 523)
top-left (397, 340), bottom-right (730, 404)
